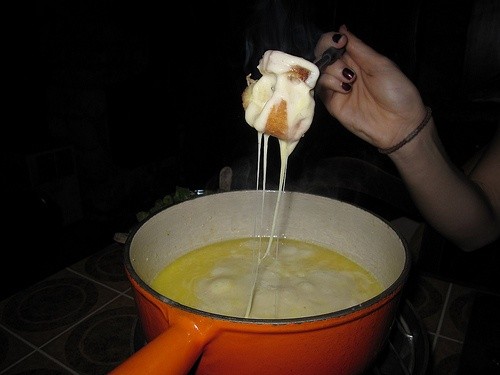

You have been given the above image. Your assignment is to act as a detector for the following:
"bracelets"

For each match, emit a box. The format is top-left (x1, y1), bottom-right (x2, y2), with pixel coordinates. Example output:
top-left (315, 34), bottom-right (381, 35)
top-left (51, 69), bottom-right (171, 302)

top-left (375, 105), bottom-right (431, 155)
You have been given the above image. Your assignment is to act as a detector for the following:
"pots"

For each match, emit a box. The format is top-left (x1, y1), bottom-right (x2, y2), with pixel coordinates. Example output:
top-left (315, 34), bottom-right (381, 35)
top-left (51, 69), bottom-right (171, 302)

top-left (101, 188), bottom-right (411, 375)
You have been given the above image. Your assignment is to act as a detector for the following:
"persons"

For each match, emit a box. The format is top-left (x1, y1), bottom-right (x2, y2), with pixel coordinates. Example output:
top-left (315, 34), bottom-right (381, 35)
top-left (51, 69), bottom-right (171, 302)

top-left (312, 24), bottom-right (500, 251)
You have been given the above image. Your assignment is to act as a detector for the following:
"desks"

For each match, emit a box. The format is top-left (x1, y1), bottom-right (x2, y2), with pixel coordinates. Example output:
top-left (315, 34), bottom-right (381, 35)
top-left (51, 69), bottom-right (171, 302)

top-left (0, 159), bottom-right (475, 375)
